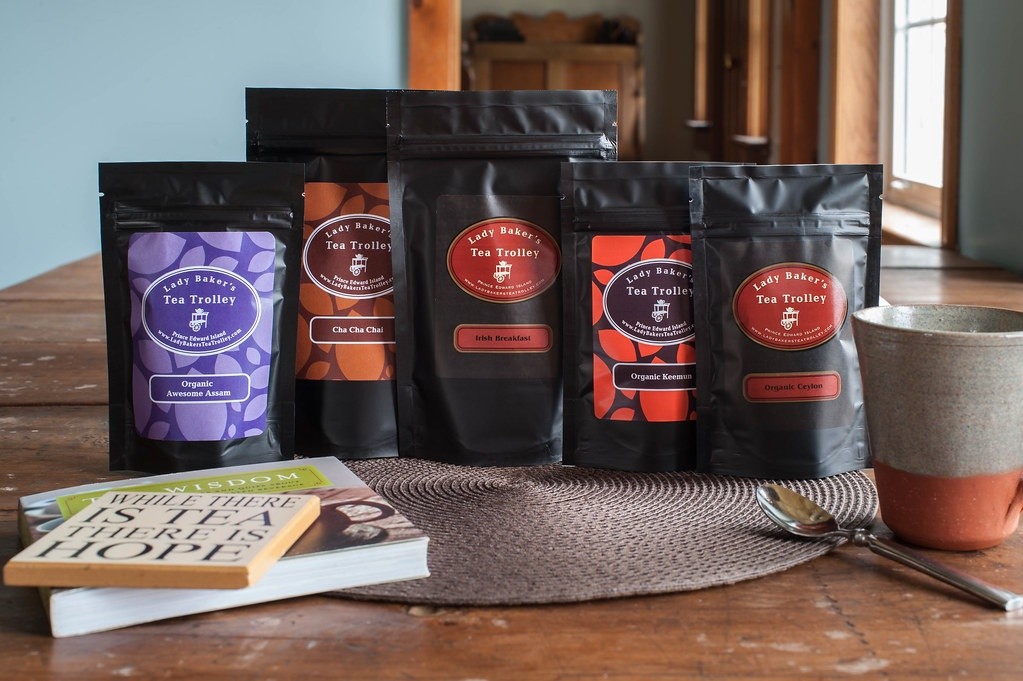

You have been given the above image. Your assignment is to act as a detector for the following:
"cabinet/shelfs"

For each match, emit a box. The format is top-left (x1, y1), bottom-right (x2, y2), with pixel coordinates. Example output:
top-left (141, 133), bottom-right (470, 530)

top-left (475, 41), bottom-right (643, 162)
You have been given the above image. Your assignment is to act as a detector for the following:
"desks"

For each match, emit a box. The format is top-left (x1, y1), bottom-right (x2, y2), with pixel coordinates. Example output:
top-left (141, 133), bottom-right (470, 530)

top-left (1, 250), bottom-right (1023, 681)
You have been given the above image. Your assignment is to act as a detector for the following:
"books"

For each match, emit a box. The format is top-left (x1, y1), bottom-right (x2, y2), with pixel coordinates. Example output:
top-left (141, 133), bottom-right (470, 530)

top-left (15, 451), bottom-right (432, 643)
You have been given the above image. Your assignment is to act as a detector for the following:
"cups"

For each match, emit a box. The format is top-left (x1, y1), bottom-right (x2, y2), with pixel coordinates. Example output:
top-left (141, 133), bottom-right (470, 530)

top-left (851, 305), bottom-right (1023, 550)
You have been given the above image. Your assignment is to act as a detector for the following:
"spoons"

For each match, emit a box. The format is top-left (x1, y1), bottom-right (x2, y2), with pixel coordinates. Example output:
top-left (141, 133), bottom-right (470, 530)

top-left (755, 484), bottom-right (1023, 611)
